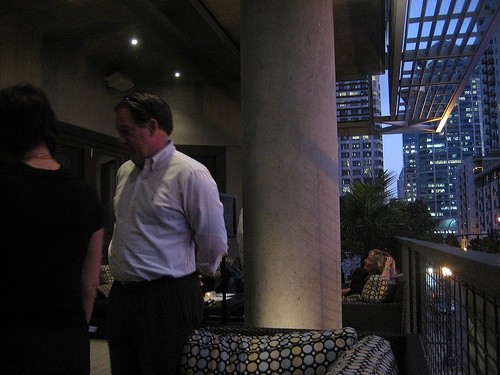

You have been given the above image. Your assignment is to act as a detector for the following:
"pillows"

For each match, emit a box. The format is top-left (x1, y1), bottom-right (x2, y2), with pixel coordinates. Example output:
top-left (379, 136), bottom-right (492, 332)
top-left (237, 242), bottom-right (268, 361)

top-left (100, 265), bottom-right (114, 284)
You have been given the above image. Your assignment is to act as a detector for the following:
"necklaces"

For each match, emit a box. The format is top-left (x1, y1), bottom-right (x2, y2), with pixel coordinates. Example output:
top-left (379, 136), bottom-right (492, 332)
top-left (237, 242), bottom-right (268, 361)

top-left (31, 154), bottom-right (54, 160)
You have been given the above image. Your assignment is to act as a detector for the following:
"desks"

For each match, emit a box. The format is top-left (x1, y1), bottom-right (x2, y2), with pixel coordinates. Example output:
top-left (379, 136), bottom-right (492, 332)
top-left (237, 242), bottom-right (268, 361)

top-left (204, 292), bottom-right (244, 326)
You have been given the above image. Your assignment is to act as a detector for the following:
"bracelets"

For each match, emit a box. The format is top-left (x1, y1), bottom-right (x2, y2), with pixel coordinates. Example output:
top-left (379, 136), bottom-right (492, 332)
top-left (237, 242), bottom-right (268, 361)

top-left (384, 266), bottom-right (389, 270)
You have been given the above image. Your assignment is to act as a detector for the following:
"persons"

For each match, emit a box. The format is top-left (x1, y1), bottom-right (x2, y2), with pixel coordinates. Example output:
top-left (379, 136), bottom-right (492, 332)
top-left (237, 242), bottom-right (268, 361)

top-left (0, 82), bottom-right (105, 375)
top-left (104, 92), bottom-right (229, 375)
top-left (342, 250), bottom-right (396, 298)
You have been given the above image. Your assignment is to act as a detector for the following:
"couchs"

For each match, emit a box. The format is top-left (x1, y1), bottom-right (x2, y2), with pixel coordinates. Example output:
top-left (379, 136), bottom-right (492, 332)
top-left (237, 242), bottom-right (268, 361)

top-left (180, 273), bottom-right (431, 375)
